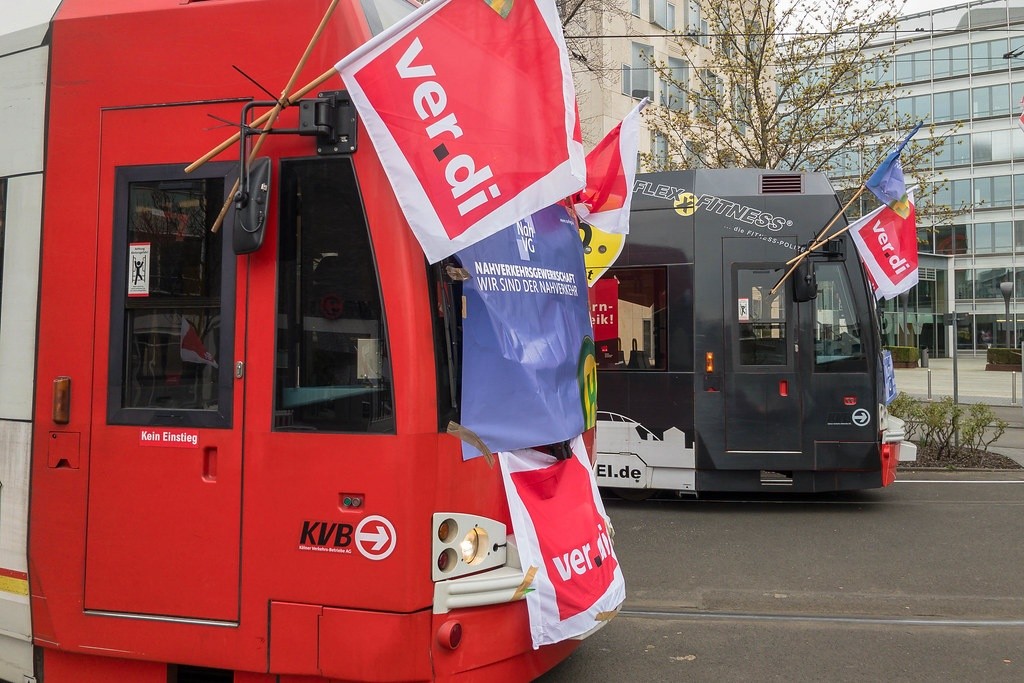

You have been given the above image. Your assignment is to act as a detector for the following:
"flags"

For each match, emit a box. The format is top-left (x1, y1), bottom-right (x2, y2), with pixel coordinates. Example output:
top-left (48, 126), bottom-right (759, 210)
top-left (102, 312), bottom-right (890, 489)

top-left (846, 118), bottom-right (925, 302)
top-left (555, 107), bottom-right (638, 235)
top-left (179, 316), bottom-right (218, 369)
top-left (332, 1), bottom-right (589, 266)
top-left (498, 432), bottom-right (625, 650)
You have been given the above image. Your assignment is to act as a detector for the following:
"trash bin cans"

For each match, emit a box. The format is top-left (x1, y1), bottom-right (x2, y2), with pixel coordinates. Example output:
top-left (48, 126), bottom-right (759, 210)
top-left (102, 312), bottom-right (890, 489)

top-left (920, 345), bottom-right (929, 368)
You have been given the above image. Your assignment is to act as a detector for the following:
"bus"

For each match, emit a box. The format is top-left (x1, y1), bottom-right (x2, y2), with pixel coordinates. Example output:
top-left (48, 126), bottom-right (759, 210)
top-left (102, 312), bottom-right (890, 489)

top-left (0, 0), bottom-right (596, 683)
top-left (582, 165), bottom-right (900, 501)
